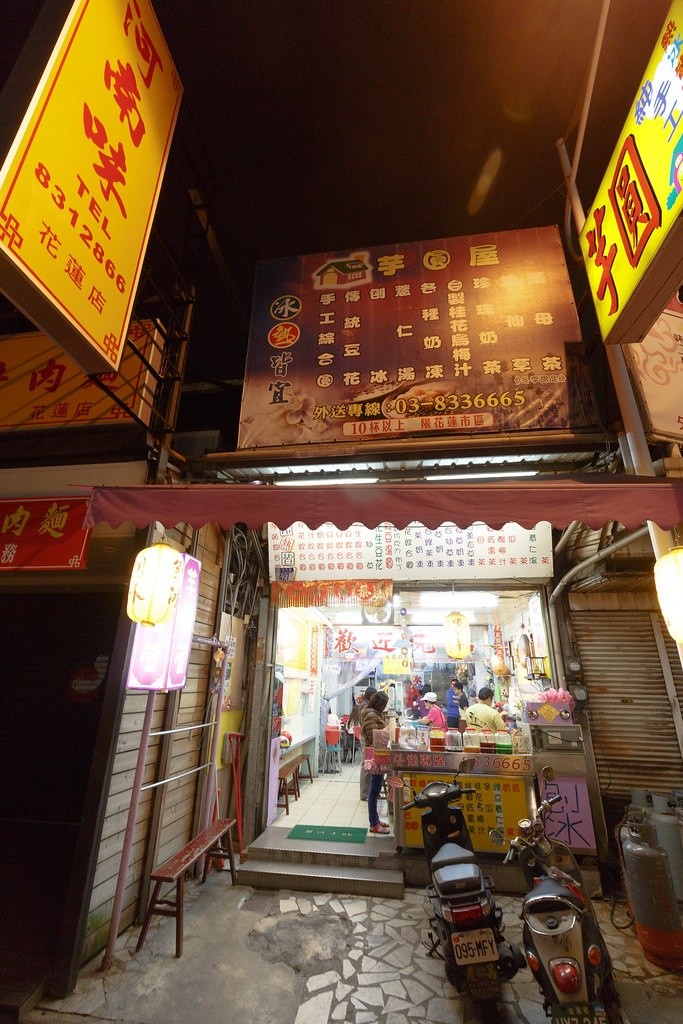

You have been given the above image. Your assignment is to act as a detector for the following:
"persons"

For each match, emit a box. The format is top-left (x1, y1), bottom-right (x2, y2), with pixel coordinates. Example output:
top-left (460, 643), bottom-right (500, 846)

top-left (404, 679), bottom-right (446, 727)
top-left (357, 687), bottom-right (376, 801)
top-left (446, 679), bottom-right (508, 733)
top-left (362, 692), bottom-right (390, 834)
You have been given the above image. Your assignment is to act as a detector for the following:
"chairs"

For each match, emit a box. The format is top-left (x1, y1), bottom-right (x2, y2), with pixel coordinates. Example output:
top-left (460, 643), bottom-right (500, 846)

top-left (324, 715), bottom-right (364, 775)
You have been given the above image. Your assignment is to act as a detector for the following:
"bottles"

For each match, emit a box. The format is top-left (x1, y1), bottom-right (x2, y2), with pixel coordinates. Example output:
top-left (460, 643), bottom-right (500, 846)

top-left (399, 720), bottom-right (417, 749)
top-left (513, 727), bottom-right (528, 754)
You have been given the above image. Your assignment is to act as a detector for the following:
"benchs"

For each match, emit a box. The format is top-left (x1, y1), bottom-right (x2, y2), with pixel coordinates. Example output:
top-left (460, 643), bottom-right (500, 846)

top-left (277, 754), bottom-right (313, 815)
top-left (135, 818), bottom-right (239, 958)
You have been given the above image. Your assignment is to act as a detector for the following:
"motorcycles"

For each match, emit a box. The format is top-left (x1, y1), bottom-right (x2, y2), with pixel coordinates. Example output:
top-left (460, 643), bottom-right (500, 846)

top-left (384, 757), bottom-right (526, 1023)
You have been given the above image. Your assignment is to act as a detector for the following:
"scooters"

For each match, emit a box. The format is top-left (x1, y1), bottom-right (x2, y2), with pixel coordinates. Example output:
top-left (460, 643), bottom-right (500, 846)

top-left (487, 765), bottom-right (630, 1024)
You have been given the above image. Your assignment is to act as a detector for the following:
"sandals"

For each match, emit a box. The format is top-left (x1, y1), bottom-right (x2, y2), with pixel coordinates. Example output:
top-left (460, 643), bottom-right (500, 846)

top-left (370, 825), bottom-right (390, 834)
top-left (376, 819), bottom-right (389, 827)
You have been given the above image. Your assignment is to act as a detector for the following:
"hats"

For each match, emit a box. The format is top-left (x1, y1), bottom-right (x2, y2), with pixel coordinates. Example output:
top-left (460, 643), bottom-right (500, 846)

top-left (420, 692), bottom-right (437, 702)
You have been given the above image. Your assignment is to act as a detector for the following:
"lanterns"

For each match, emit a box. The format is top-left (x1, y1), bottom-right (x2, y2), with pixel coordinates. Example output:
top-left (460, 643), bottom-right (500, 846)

top-left (654, 546), bottom-right (683, 641)
top-left (127, 544), bottom-right (185, 626)
top-left (443, 612), bottom-right (470, 660)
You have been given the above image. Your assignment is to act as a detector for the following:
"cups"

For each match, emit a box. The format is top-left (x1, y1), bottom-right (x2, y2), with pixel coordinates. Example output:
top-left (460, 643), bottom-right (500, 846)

top-left (417, 725), bottom-right (430, 751)
